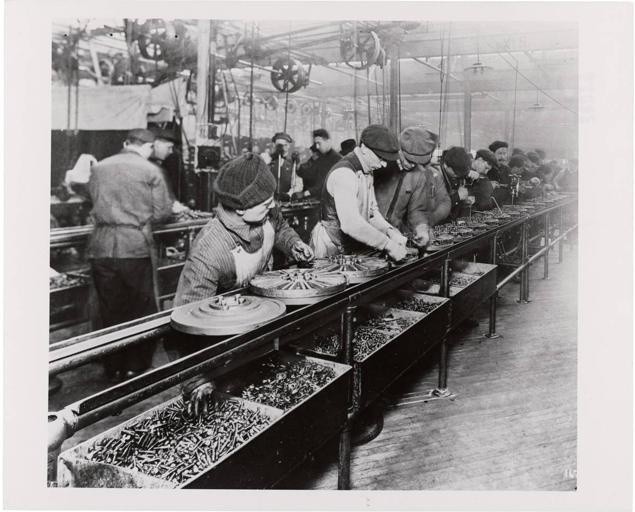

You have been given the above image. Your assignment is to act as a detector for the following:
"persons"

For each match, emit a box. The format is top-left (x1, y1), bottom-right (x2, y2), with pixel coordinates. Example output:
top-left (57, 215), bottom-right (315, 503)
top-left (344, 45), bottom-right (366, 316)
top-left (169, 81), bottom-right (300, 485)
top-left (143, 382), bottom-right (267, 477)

top-left (148, 128), bottom-right (190, 223)
top-left (85, 128), bottom-right (175, 384)
top-left (159, 150), bottom-right (316, 417)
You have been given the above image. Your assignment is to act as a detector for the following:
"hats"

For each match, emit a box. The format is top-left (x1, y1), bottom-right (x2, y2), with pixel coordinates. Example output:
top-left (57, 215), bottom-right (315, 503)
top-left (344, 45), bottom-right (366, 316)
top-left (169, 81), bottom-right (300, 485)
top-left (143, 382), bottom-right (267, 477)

top-left (477, 150), bottom-right (500, 170)
top-left (444, 147), bottom-right (470, 176)
top-left (488, 141), bottom-right (509, 151)
top-left (126, 128), bottom-right (178, 142)
top-left (398, 126), bottom-right (435, 164)
top-left (271, 133), bottom-right (292, 143)
top-left (213, 152), bottom-right (277, 210)
top-left (339, 139), bottom-right (357, 155)
top-left (360, 124), bottom-right (401, 160)
